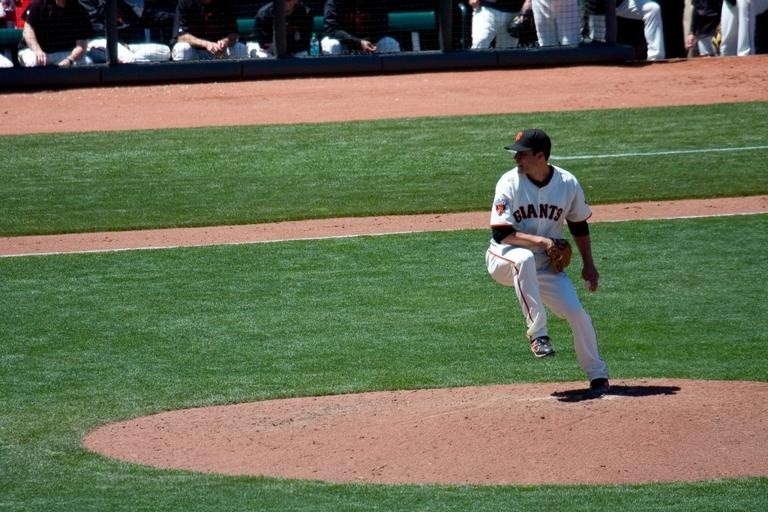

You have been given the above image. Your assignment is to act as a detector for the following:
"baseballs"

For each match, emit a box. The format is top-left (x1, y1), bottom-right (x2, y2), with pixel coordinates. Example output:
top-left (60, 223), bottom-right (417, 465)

top-left (585, 280), bottom-right (592, 292)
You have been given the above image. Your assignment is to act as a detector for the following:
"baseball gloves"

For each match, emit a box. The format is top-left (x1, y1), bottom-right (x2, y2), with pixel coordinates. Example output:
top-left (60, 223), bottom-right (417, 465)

top-left (544, 237), bottom-right (572, 274)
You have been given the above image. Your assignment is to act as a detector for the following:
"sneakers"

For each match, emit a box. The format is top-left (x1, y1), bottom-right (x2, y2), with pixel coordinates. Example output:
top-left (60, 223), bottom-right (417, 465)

top-left (590, 378), bottom-right (610, 395)
top-left (530, 337), bottom-right (555, 359)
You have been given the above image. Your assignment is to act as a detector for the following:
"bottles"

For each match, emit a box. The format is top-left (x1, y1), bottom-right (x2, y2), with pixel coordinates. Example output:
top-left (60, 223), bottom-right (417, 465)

top-left (310, 33), bottom-right (319, 58)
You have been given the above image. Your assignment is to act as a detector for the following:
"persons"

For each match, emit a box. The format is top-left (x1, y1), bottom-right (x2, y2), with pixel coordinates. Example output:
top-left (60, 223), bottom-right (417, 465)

top-left (79, 0), bottom-right (249, 63)
top-left (469, 1), bottom-right (665, 60)
top-left (484, 128), bottom-right (611, 398)
top-left (320, 0), bottom-right (400, 56)
top-left (247, 1), bottom-right (313, 59)
top-left (1, 0), bottom-right (94, 66)
top-left (683, 0), bottom-right (768, 58)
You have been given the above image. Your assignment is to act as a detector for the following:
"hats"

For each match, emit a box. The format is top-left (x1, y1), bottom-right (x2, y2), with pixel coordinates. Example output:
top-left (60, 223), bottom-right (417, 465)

top-left (502, 127), bottom-right (552, 156)
